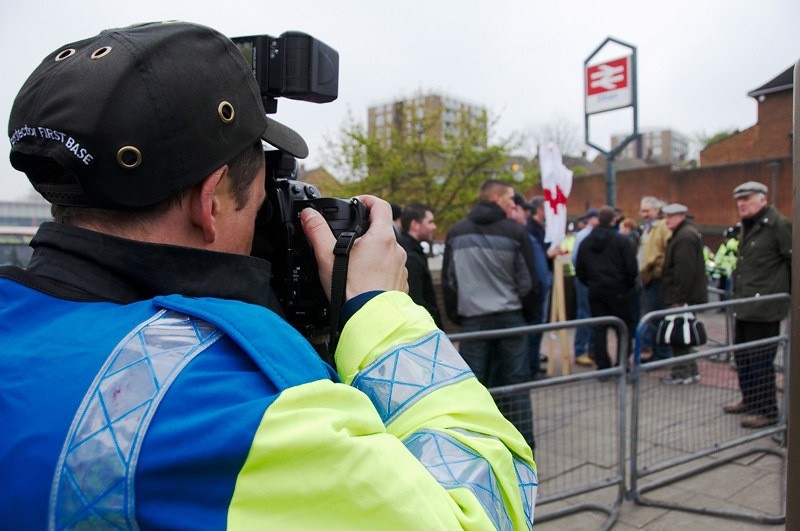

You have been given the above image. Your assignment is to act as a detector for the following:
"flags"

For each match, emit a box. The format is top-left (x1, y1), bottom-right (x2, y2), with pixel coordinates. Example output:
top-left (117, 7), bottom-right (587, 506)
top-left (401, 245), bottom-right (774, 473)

top-left (539, 143), bottom-right (574, 245)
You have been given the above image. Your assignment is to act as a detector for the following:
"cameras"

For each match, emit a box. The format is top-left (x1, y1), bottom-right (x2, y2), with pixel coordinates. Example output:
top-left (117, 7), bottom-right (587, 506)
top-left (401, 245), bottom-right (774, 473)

top-left (248, 146), bottom-right (403, 340)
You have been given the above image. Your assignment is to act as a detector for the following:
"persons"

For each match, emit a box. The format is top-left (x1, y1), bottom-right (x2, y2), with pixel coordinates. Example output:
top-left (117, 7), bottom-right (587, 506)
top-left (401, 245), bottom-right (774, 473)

top-left (723, 182), bottom-right (793, 428)
top-left (389, 180), bottom-right (741, 461)
top-left (0, 21), bottom-right (537, 531)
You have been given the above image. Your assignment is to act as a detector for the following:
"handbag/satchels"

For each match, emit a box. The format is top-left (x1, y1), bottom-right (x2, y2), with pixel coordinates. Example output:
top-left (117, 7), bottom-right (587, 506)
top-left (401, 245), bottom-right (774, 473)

top-left (656, 312), bottom-right (706, 345)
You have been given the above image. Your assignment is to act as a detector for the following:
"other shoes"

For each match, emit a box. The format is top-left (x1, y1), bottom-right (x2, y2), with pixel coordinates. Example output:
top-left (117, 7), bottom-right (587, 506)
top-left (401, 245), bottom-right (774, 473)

top-left (724, 400), bottom-right (757, 413)
top-left (741, 413), bottom-right (779, 427)
top-left (576, 353), bottom-right (594, 366)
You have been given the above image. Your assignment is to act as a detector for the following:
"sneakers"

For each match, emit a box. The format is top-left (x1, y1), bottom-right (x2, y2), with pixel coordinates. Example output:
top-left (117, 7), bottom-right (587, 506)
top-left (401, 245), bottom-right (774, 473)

top-left (660, 374), bottom-right (701, 384)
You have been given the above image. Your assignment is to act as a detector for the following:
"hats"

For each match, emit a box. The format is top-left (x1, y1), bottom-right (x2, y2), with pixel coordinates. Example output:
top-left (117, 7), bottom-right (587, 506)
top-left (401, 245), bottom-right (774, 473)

top-left (512, 191), bottom-right (536, 210)
top-left (661, 203), bottom-right (688, 213)
top-left (10, 20), bottom-right (308, 209)
top-left (587, 208), bottom-right (599, 216)
top-left (733, 181), bottom-right (768, 198)
